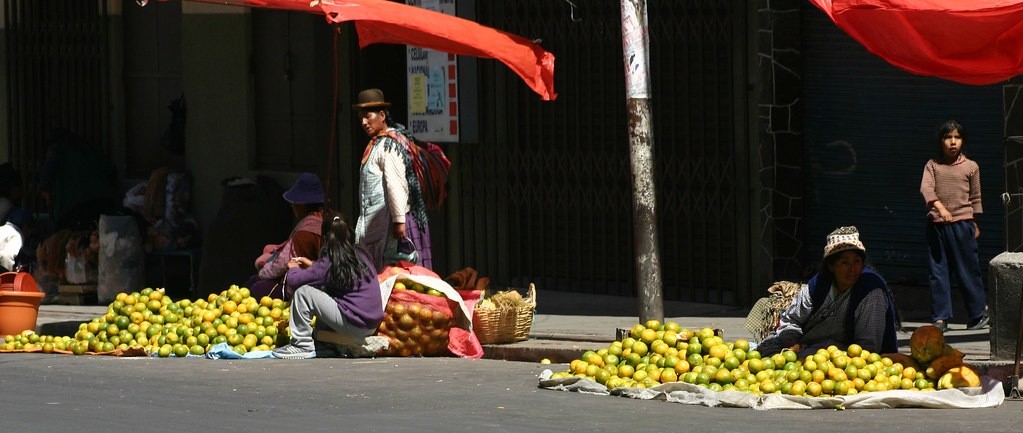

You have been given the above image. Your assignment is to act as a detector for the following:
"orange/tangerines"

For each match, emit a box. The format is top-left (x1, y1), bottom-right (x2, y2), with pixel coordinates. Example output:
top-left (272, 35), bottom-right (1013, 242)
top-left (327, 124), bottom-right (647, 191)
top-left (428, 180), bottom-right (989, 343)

top-left (377, 279), bottom-right (457, 355)
top-left (0, 288), bottom-right (317, 356)
top-left (546, 316), bottom-right (938, 396)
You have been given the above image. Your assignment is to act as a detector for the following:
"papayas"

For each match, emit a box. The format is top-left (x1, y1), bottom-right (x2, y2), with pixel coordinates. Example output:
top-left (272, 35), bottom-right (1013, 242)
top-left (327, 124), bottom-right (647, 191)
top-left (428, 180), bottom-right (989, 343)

top-left (879, 325), bottom-right (982, 390)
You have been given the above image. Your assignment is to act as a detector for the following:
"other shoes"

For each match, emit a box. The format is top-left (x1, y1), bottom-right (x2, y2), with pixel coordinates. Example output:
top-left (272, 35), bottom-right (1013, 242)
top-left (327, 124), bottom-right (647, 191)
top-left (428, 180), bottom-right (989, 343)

top-left (934, 320), bottom-right (948, 333)
top-left (967, 314), bottom-right (990, 330)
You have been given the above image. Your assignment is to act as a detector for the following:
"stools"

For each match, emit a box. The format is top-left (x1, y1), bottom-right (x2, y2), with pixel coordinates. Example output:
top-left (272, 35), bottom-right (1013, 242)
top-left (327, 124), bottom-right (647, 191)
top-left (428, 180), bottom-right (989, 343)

top-left (59, 284), bottom-right (97, 307)
top-left (151, 249), bottom-right (200, 299)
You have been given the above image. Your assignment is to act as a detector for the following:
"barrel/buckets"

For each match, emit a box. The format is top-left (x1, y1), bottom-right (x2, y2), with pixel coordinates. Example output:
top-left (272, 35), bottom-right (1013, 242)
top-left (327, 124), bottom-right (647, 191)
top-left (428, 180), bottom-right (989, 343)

top-left (456, 290), bottom-right (486, 319)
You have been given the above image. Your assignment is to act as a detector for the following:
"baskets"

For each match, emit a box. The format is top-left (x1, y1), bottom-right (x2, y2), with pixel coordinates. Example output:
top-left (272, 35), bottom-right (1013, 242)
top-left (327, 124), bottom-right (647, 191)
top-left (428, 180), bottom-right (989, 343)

top-left (473, 283), bottom-right (536, 344)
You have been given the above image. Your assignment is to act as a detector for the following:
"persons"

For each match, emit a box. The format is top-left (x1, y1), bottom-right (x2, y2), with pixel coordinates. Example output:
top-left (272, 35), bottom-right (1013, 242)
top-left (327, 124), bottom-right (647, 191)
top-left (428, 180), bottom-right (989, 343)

top-left (354, 88), bottom-right (431, 270)
top-left (240, 172), bottom-right (384, 358)
top-left (0, 127), bottom-right (117, 272)
top-left (761, 226), bottom-right (901, 357)
top-left (918, 120), bottom-right (990, 332)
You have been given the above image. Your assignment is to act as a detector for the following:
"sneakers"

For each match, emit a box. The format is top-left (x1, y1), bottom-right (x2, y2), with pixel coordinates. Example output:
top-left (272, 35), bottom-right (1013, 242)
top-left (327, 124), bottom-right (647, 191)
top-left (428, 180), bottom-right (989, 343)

top-left (272, 344), bottom-right (316, 359)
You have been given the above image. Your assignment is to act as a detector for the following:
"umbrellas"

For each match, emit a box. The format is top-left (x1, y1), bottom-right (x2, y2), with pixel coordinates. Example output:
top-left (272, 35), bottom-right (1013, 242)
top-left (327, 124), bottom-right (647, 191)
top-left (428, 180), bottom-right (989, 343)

top-left (202, 0), bottom-right (557, 212)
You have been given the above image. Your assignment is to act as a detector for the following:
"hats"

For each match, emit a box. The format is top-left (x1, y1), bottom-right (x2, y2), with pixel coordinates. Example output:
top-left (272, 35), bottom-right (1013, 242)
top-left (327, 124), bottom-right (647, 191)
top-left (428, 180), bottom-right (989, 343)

top-left (283, 171), bottom-right (331, 204)
top-left (824, 225), bottom-right (866, 258)
top-left (353, 89), bottom-right (391, 108)
